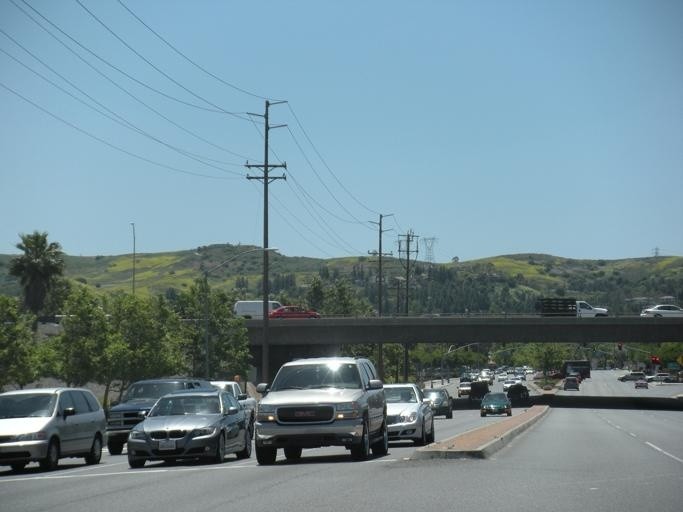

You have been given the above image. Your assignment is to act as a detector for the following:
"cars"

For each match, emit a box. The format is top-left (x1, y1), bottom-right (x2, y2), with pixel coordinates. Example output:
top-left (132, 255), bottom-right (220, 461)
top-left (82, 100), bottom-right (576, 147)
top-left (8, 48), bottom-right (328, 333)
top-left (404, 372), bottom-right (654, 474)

top-left (640, 302), bottom-right (681, 318)
top-left (563, 370), bottom-right (672, 389)
top-left (267, 306), bottom-right (318, 320)
top-left (457, 366), bottom-right (528, 418)
top-left (126, 390), bottom-right (252, 465)
top-left (421, 386), bottom-right (453, 421)
top-left (381, 382), bottom-right (435, 444)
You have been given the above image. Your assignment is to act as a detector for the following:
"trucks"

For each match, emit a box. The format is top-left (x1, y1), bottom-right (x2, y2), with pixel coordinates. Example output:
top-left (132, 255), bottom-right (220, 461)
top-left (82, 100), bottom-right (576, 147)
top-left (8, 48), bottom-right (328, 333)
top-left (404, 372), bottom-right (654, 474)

top-left (536, 297), bottom-right (608, 318)
top-left (233, 301), bottom-right (280, 318)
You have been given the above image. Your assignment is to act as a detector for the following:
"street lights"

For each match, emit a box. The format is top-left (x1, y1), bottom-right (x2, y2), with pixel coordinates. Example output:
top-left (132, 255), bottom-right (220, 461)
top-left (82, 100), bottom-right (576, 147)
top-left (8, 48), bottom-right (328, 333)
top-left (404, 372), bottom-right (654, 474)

top-left (201, 247), bottom-right (280, 376)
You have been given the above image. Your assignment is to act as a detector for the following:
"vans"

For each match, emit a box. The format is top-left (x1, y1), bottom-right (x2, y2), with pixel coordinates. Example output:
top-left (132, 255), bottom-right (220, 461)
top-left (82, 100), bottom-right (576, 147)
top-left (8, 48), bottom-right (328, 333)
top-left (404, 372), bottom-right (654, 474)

top-left (0, 387), bottom-right (101, 471)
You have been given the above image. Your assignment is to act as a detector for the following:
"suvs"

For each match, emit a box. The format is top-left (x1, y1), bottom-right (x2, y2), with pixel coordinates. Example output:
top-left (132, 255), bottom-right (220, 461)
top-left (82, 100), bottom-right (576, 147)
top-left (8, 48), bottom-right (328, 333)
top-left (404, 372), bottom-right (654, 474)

top-left (106, 378), bottom-right (206, 454)
top-left (254, 355), bottom-right (387, 464)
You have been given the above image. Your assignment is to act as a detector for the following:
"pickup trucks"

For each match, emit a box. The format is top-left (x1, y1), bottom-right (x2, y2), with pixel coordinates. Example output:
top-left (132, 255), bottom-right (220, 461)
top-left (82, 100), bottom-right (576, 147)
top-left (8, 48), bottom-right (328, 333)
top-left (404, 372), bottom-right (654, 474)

top-left (208, 380), bottom-right (255, 442)
